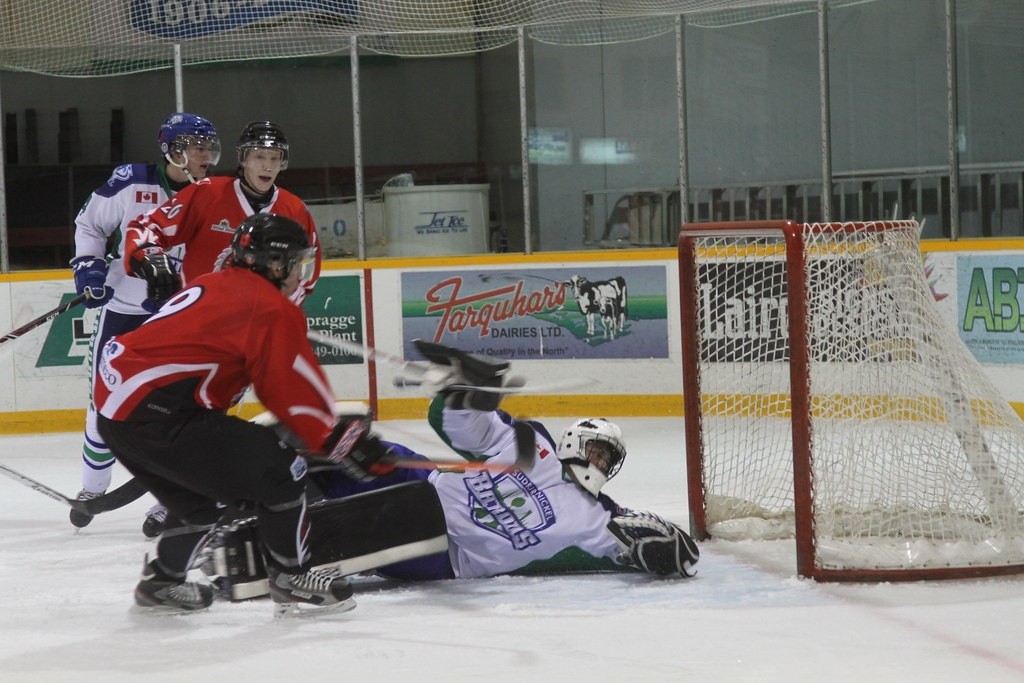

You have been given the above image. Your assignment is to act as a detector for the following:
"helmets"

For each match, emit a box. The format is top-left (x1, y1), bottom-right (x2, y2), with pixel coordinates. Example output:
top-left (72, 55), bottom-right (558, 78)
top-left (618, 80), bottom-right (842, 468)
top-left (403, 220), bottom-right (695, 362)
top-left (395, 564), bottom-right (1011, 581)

top-left (158, 112), bottom-right (217, 154)
top-left (230, 213), bottom-right (312, 283)
top-left (235, 121), bottom-right (290, 151)
top-left (555, 416), bottom-right (627, 499)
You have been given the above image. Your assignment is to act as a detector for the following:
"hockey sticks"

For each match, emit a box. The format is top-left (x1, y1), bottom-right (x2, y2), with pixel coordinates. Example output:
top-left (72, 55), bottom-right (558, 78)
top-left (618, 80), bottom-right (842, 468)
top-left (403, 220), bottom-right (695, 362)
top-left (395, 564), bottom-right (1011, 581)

top-left (301, 420), bottom-right (538, 474)
top-left (0, 461), bottom-right (151, 516)
top-left (306, 325), bottom-right (431, 382)
top-left (0, 289), bottom-right (93, 349)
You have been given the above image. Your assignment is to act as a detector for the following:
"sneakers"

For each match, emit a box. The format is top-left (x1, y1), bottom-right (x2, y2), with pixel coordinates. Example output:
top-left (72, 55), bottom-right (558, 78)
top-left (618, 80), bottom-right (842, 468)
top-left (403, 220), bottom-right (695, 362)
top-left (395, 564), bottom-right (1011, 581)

top-left (254, 530), bottom-right (361, 618)
top-left (69, 488), bottom-right (107, 536)
top-left (134, 558), bottom-right (216, 615)
top-left (141, 502), bottom-right (170, 543)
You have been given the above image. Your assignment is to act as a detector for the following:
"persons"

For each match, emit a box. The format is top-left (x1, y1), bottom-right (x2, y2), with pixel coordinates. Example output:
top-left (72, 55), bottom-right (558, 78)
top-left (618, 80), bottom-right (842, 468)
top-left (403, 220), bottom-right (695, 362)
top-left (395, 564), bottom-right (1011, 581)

top-left (94, 211), bottom-right (371, 621)
top-left (70, 113), bottom-right (700, 602)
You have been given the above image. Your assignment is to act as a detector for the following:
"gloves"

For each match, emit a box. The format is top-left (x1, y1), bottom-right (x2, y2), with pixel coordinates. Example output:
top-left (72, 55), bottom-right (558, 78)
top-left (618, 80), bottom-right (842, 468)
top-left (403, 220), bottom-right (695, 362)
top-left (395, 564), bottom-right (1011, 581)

top-left (69, 254), bottom-right (115, 309)
top-left (140, 253), bottom-right (183, 315)
top-left (319, 413), bottom-right (399, 484)
